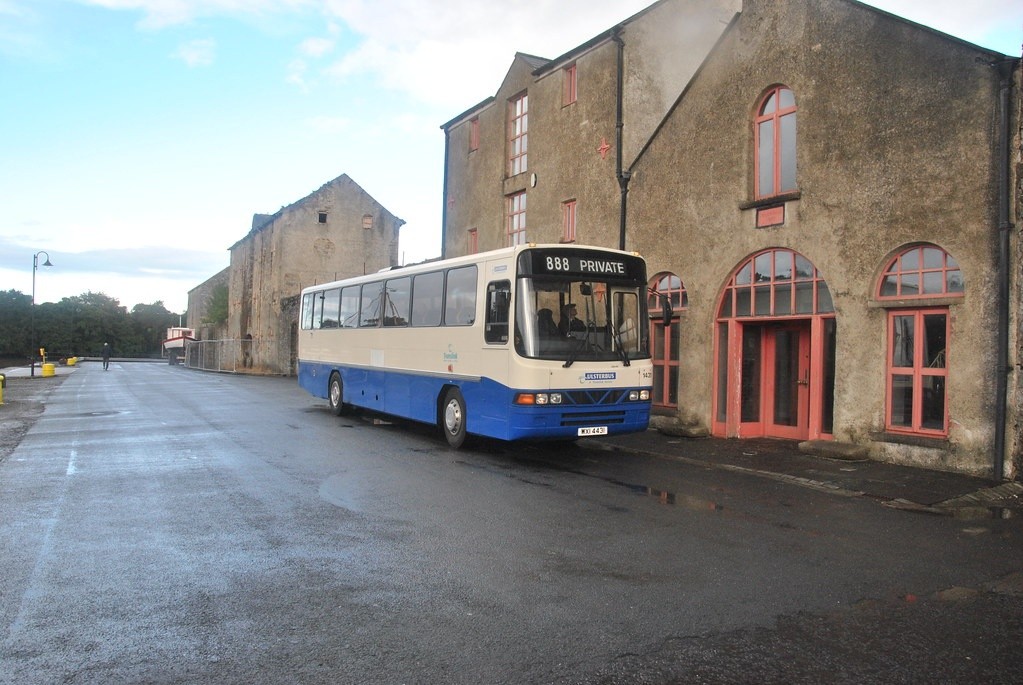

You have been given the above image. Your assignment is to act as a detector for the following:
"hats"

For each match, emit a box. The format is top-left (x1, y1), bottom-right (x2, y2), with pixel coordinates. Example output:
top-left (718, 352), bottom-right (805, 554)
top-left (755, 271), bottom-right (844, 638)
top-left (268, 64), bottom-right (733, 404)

top-left (104, 343), bottom-right (108, 345)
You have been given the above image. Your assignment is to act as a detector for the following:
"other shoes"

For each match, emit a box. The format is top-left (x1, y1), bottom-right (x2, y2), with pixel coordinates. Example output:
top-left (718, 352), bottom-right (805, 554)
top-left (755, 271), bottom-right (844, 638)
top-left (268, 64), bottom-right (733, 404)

top-left (103, 368), bottom-right (105, 369)
top-left (105, 369), bottom-right (107, 371)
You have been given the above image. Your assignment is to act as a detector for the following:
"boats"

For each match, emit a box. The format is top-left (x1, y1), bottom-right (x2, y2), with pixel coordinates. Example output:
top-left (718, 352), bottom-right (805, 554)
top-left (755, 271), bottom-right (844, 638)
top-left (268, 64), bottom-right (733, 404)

top-left (162, 310), bottom-right (198, 357)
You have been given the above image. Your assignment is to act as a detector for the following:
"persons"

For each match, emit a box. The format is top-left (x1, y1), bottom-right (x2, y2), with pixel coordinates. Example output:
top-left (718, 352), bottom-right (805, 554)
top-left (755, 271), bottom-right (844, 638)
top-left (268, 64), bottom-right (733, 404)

top-left (101, 343), bottom-right (113, 371)
top-left (559, 304), bottom-right (586, 335)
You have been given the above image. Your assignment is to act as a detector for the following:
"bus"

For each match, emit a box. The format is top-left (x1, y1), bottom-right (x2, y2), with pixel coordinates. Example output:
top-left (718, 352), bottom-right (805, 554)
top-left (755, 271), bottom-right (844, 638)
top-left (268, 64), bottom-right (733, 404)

top-left (294, 242), bottom-right (673, 449)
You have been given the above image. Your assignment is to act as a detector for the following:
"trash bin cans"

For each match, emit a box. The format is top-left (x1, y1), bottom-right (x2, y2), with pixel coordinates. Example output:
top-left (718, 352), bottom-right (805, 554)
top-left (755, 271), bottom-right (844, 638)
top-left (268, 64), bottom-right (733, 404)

top-left (169, 355), bottom-right (177, 365)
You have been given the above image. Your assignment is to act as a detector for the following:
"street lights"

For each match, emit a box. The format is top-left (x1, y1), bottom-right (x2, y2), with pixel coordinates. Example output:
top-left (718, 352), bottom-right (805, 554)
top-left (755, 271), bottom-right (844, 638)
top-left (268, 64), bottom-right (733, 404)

top-left (30, 251), bottom-right (54, 378)
